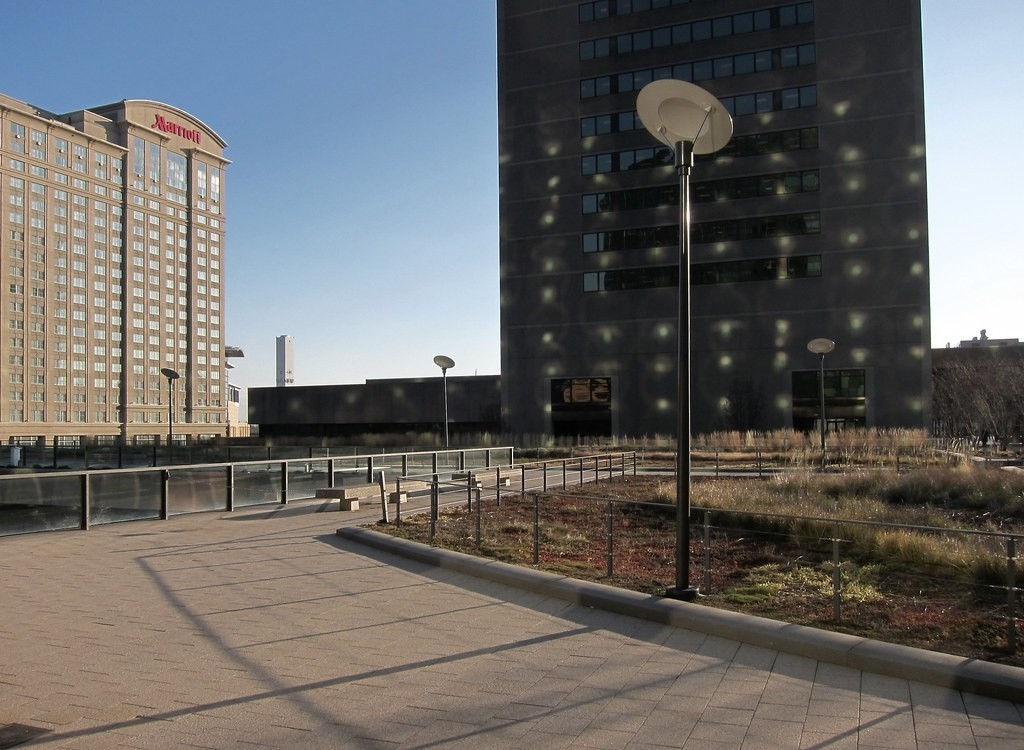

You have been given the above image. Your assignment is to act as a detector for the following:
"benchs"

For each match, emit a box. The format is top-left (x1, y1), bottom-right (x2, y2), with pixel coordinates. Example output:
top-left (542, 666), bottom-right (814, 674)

top-left (316, 480), bottom-right (426, 511)
top-left (452, 468), bottom-right (522, 491)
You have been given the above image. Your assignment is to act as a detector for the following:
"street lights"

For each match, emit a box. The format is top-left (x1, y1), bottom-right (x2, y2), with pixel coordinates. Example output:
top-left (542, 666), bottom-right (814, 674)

top-left (636, 76), bottom-right (734, 608)
top-left (161, 368), bottom-right (180, 464)
top-left (434, 355), bottom-right (455, 464)
top-left (807, 338), bottom-right (835, 452)
